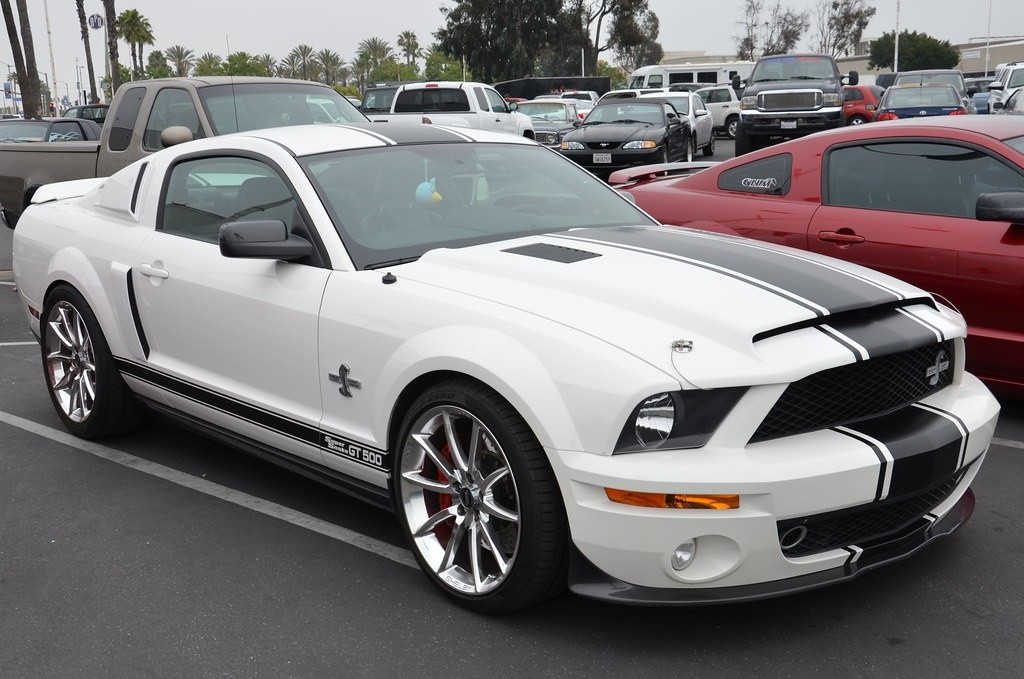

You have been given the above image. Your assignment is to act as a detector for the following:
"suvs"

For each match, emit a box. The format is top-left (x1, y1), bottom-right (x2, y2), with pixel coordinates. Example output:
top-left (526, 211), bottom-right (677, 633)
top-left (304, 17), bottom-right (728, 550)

top-left (731, 54), bottom-right (859, 157)
top-left (361, 81), bottom-right (402, 122)
top-left (693, 82), bottom-right (746, 139)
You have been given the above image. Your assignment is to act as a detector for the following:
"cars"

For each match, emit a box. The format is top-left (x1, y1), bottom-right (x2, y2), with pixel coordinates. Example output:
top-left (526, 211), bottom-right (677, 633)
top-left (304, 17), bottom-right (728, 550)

top-left (987, 61), bottom-right (1024, 114)
top-left (842, 84), bottom-right (900, 126)
top-left (637, 92), bottom-right (715, 156)
top-left (609, 115), bottom-right (1024, 403)
top-left (995, 89), bottom-right (1024, 115)
top-left (594, 89), bottom-right (664, 107)
top-left (513, 99), bottom-right (580, 152)
top-left (893, 70), bottom-right (977, 115)
top-left (61, 105), bottom-right (109, 128)
top-left (963, 76), bottom-right (996, 115)
top-left (1, 117), bottom-right (102, 143)
top-left (10, 122), bottom-right (1002, 615)
top-left (866, 83), bottom-right (969, 122)
top-left (560, 98), bottom-right (694, 176)
top-left (560, 91), bottom-right (600, 122)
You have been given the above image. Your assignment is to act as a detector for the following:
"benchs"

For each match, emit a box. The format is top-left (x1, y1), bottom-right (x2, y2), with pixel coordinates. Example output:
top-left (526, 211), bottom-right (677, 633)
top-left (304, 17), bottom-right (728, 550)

top-left (188, 186), bottom-right (356, 240)
top-left (618, 112), bottom-right (661, 122)
top-left (892, 95), bottom-right (949, 106)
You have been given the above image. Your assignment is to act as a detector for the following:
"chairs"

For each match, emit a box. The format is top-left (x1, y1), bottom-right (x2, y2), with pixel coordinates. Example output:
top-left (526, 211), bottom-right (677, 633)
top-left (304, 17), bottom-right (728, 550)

top-left (766, 72), bottom-right (780, 79)
top-left (944, 155), bottom-right (1000, 217)
top-left (253, 106), bottom-right (287, 130)
top-left (815, 64), bottom-right (832, 74)
top-left (362, 170), bottom-right (432, 235)
top-left (847, 166), bottom-right (912, 212)
top-left (715, 95), bottom-right (721, 102)
top-left (600, 108), bottom-right (618, 122)
top-left (643, 107), bottom-right (659, 122)
top-left (176, 105), bottom-right (218, 133)
top-left (235, 177), bottom-right (311, 244)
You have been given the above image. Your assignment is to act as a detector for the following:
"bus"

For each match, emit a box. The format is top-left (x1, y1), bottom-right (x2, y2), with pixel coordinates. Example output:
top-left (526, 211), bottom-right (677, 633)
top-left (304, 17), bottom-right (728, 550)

top-left (627, 60), bottom-right (757, 89)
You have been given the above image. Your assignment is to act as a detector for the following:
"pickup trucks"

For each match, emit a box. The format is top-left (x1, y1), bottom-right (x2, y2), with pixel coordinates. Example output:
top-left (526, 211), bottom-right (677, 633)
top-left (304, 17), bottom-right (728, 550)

top-left (0, 76), bottom-right (375, 229)
top-left (389, 81), bottom-right (536, 141)
